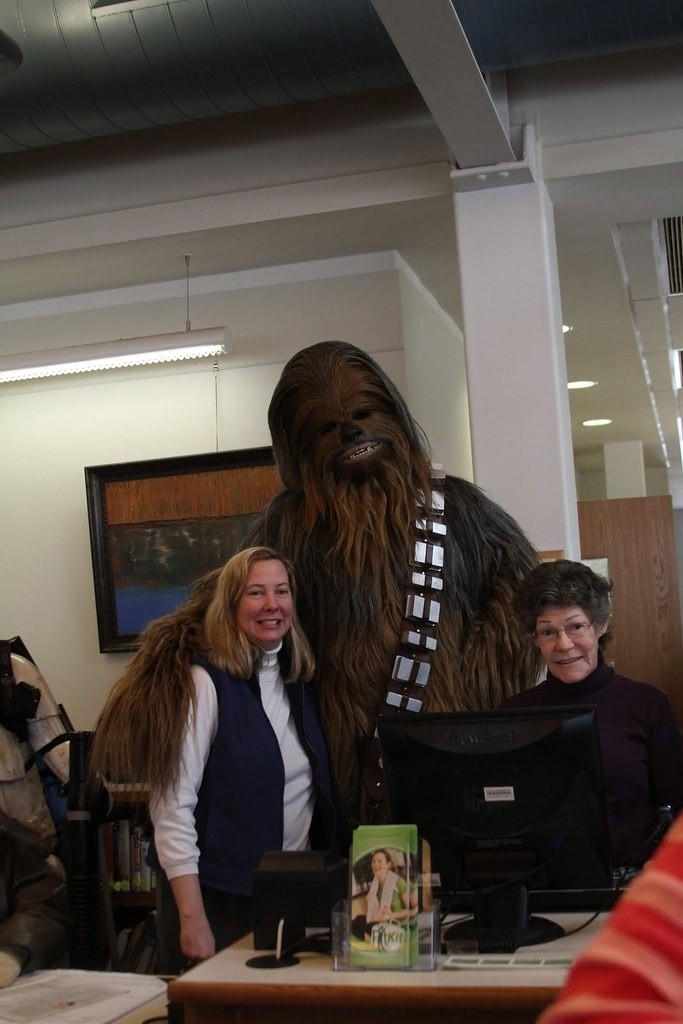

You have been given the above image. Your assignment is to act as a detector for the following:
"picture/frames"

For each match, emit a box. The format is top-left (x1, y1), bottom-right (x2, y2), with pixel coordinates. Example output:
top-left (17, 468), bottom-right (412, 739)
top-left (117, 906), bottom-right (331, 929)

top-left (84, 443), bottom-right (317, 663)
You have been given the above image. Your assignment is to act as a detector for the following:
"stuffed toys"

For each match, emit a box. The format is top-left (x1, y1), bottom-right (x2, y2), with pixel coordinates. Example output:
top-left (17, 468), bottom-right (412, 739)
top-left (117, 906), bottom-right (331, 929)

top-left (95, 342), bottom-right (552, 822)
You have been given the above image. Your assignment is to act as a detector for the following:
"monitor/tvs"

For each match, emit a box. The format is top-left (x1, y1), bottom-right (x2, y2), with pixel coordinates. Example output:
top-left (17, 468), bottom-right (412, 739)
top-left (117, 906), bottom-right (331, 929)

top-left (378, 704), bottom-right (618, 912)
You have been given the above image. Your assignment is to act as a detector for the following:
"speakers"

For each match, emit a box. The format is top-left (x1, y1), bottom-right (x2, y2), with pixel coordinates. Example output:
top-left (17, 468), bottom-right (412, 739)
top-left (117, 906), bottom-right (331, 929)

top-left (251, 851), bottom-right (349, 951)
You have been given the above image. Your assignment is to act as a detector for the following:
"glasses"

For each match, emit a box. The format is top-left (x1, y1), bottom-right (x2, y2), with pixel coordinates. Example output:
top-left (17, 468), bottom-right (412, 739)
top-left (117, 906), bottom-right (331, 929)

top-left (532, 621), bottom-right (595, 642)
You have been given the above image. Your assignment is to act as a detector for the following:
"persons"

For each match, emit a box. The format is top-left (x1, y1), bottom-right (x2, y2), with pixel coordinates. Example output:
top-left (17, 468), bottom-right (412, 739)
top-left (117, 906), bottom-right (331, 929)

top-left (92, 549), bottom-right (330, 979)
top-left (351, 849), bottom-right (417, 947)
top-left (439, 562), bottom-right (683, 907)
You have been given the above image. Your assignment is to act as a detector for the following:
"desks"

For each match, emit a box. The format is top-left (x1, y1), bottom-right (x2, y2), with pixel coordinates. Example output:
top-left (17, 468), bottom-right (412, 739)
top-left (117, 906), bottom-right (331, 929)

top-left (163, 868), bottom-right (609, 1023)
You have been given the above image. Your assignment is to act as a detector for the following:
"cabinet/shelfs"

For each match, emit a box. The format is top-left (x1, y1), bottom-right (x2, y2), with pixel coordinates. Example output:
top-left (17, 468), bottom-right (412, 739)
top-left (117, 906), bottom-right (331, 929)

top-left (72, 725), bottom-right (225, 978)
